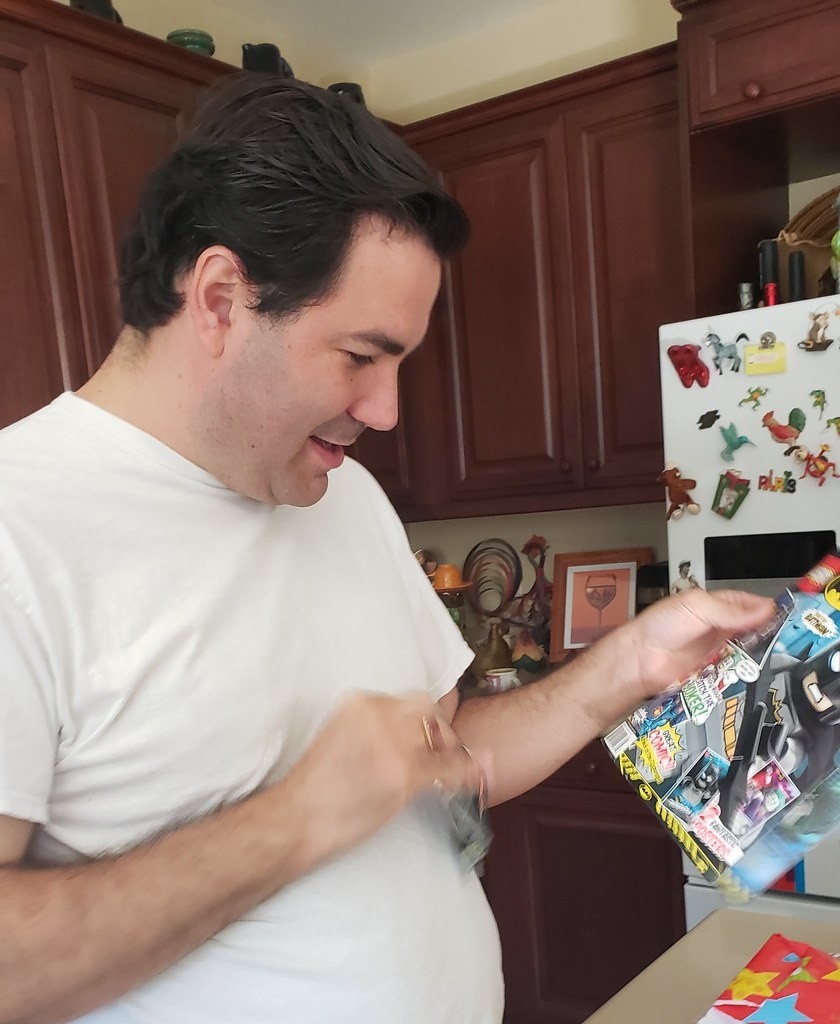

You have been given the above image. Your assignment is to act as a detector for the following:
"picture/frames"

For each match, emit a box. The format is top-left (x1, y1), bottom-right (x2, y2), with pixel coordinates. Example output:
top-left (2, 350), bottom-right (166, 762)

top-left (548, 551), bottom-right (636, 654)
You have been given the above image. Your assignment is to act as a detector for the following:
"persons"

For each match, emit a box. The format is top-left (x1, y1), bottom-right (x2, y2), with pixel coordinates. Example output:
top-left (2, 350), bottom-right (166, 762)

top-left (0, 78), bottom-right (775, 1024)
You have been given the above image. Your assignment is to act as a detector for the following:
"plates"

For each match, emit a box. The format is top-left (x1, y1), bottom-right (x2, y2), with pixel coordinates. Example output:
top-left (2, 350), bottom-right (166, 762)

top-left (431, 581), bottom-right (473, 591)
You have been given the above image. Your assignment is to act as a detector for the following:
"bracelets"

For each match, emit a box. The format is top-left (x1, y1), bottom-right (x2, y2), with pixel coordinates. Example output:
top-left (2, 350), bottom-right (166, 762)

top-left (424, 719), bottom-right (439, 749)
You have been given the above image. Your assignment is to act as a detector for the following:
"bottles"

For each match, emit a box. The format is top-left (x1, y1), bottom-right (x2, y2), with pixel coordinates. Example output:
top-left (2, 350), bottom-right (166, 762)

top-left (765, 282), bottom-right (776, 306)
top-left (739, 283), bottom-right (754, 310)
top-left (788, 250), bottom-right (805, 301)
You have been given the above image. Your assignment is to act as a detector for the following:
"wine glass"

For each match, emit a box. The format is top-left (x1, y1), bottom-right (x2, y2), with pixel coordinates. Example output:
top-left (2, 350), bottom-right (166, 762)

top-left (584, 574), bottom-right (616, 640)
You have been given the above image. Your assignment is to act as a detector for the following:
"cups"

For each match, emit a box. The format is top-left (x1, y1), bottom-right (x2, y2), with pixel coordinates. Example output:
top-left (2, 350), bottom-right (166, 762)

top-left (487, 668), bottom-right (522, 695)
top-left (434, 565), bottom-right (460, 586)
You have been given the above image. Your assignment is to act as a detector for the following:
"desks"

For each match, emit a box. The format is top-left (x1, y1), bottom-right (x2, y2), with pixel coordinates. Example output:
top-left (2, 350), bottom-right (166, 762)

top-left (582, 886), bottom-right (839, 1024)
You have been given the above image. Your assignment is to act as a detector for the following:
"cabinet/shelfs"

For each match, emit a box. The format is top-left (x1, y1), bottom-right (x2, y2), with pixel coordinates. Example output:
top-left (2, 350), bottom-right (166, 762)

top-left (0, 0), bottom-right (789, 530)
top-left (477, 734), bottom-right (687, 1024)
top-left (673, 1), bottom-right (840, 136)
